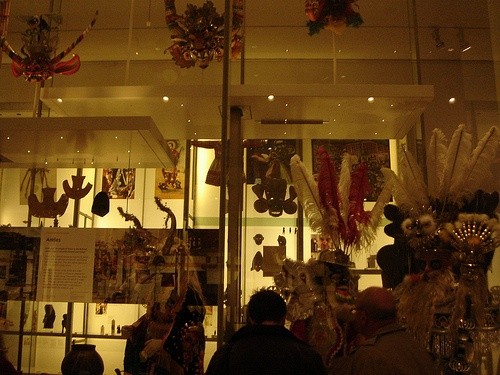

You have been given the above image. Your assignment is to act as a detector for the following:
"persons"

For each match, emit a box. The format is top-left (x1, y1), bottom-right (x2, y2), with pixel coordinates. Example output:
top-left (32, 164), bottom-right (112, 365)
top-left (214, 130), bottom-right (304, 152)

top-left (205, 289), bottom-right (325, 375)
top-left (351, 287), bottom-right (436, 375)
top-left (124, 301), bottom-right (183, 374)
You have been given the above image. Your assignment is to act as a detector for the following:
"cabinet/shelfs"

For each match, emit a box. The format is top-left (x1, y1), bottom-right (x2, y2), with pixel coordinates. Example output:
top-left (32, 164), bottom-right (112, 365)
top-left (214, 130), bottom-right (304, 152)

top-left (233, 136), bottom-right (402, 333)
top-left (0, 168), bottom-right (224, 375)
